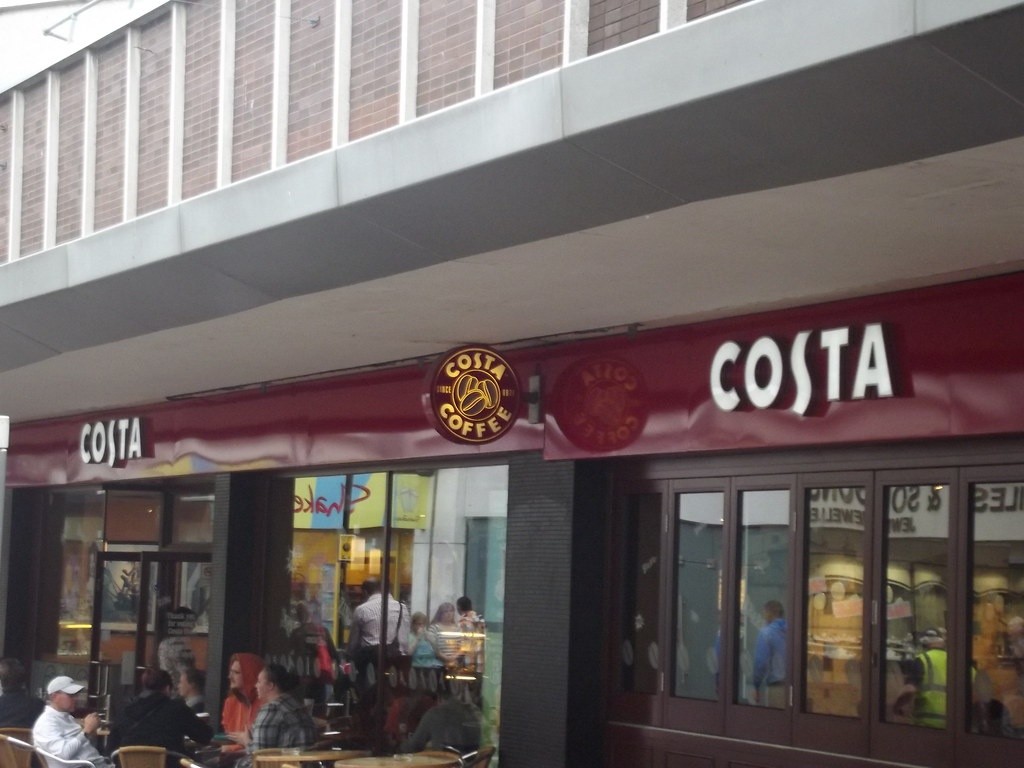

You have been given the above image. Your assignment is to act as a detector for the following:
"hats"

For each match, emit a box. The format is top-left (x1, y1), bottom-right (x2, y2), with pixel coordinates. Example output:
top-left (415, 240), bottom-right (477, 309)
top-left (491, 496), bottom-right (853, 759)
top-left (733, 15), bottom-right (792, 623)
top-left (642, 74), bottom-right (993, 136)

top-left (47, 676), bottom-right (85, 695)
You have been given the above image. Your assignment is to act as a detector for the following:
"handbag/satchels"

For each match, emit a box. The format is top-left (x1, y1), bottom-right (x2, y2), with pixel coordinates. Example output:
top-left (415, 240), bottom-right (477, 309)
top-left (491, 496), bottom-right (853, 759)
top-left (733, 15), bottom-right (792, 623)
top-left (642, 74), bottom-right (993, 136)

top-left (358, 643), bottom-right (402, 669)
top-left (313, 622), bottom-right (332, 681)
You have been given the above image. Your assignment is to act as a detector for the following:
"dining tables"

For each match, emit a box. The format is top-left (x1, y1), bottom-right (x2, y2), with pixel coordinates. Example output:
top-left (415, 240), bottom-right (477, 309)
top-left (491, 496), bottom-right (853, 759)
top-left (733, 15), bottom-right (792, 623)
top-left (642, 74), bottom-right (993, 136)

top-left (256, 750), bottom-right (372, 768)
top-left (334, 755), bottom-right (458, 767)
top-left (806, 633), bottom-right (921, 689)
top-left (185, 736), bottom-right (237, 768)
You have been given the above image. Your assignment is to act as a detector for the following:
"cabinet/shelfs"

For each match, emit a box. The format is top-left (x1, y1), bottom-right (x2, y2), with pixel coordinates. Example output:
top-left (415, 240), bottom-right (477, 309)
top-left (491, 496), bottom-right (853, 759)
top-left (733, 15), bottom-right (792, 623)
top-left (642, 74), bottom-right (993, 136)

top-left (436, 615), bottom-right (485, 710)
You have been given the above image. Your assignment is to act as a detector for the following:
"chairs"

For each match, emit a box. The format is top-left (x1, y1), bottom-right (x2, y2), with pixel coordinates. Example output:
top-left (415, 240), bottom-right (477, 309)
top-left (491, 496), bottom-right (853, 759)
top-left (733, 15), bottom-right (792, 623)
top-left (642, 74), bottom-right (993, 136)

top-left (1, 618), bottom-right (497, 768)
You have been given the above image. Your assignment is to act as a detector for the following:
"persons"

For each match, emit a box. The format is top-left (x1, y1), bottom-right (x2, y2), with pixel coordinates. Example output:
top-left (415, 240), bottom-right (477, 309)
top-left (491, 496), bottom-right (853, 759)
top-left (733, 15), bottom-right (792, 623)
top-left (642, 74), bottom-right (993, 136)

top-left (404, 612), bottom-right (442, 702)
top-left (285, 600), bottom-right (340, 744)
top-left (349, 576), bottom-right (412, 699)
top-left (220, 651), bottom-right (267, 768)
top-left (457, 597), bottom-right (485, 712)
top-left (236, 661), bottom-right (319, 768)
top-left (156, 607), bottom-right (196, 690)
top-left (892, 632), bottom-right (992, 734)
top-left (753, 601), bottom-right (792, 710)
top-left (424, 602), bottom-right (463, 704)
top-left (31, 675), bottom-right (117, 768)
top-left (175, 667), bottom-right (205, 713)
top-left (0, 656), bottom-right (45, 727)
top-left (105, 666), bottom-right (215, 768)
